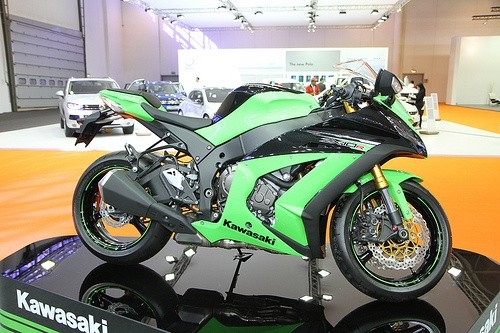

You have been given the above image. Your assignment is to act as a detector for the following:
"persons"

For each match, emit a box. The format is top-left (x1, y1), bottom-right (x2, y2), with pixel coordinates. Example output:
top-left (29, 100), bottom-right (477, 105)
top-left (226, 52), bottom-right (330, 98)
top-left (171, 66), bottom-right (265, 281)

top-left (306, 78), bottom-right (326, 96)
top-left (411, 80), bottom-right (425, 128)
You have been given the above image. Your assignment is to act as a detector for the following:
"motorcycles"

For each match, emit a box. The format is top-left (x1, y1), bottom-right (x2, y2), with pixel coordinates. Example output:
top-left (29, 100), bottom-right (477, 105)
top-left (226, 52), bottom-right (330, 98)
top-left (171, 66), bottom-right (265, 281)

top-left (71, 70), bottom-right (455, 302)
top-left (79, 253), bottom-right (446, 333)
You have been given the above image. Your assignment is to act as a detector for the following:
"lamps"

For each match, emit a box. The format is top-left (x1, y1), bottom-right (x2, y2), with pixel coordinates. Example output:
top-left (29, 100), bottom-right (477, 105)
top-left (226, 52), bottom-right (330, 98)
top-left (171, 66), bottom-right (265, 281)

top-left (171, 20), bottom-right (178, 25)
top-left (145, 7), bottom-right (153, 13)
top-left (305, 2), bottom-right (317, 33)
top-left (370, 9), bottom-right (389, 32)
top-left (161, 16), bottom-right (169, 21)
top-left (177, 14), bottom-right (185, 19)
top-left (338, 10), bottom-right (347, 15)
top-left (216, 5), bottom-right (253, 35)
top-left (254, 10), bottom-right (263, 16)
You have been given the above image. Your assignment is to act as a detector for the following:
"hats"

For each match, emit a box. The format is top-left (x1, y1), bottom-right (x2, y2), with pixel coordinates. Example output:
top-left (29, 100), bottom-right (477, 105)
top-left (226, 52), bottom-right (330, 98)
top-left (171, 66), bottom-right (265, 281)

top-left (311, 78), bottom-right (318, 82)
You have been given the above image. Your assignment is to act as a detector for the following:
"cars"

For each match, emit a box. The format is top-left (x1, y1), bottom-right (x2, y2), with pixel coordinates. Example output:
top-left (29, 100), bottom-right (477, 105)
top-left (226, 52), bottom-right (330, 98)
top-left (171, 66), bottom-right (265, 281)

top-left (394, 93), bottom-right (421, 128)
top-left (56, 74), bottom-right (135, 138)
top-left (124, 79), bottom-right (186, 112)
top-left (178, 87), bottom-right (234, 118)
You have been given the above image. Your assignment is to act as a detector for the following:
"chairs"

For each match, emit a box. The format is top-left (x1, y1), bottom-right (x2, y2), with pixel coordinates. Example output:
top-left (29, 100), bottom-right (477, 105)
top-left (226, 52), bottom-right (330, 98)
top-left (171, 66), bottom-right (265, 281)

top-left (71, 82), bottom-right (82, 94)
